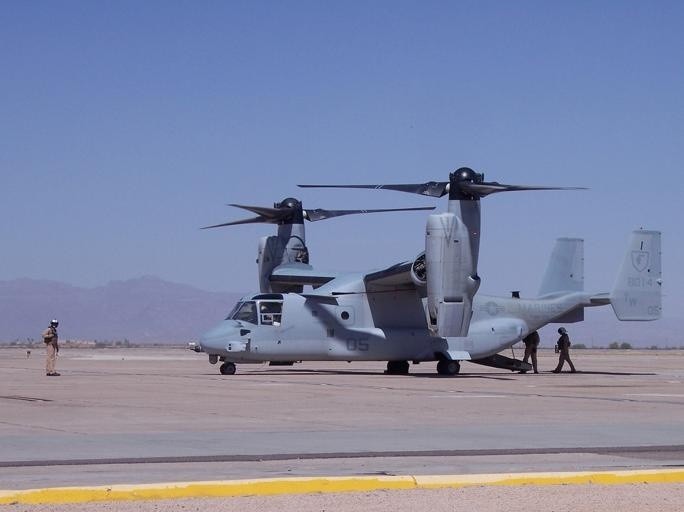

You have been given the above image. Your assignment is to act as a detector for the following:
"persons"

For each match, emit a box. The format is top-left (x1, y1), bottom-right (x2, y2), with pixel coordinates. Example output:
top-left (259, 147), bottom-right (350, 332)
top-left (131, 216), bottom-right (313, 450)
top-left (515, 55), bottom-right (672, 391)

top-left (41, 318), bottom-right (60, 376)
top-left (518, 331), bottom-right (540, 373)
top-left (552, 327), bottom-right (576, 373)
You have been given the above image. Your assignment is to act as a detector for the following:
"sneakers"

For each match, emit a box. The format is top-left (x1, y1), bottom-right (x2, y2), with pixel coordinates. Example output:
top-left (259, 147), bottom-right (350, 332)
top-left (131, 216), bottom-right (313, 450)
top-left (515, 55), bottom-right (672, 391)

top-left (570, 369), bottom-right (576, 373)
top-left (46, 372), bottom-right (61, 376)
top-left (553, 370), bottom-right (561, 373)
top-left (518, 371), bottom-right (526, 374)
top-left (534, 371), bottom-right (539, 374)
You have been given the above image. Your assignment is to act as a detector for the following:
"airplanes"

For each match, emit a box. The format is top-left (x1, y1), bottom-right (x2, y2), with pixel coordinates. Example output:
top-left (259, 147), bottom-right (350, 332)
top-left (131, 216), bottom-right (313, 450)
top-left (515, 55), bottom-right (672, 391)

top-left (190, 166), bottom-right (662, 376)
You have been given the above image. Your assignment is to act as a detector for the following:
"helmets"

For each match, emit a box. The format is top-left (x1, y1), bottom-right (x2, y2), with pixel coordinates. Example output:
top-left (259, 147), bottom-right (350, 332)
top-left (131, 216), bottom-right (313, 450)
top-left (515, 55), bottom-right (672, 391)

top-left (50, 319), bottom-right (59, 328)
top-left (558, 327), bottom-right (566, 334)
